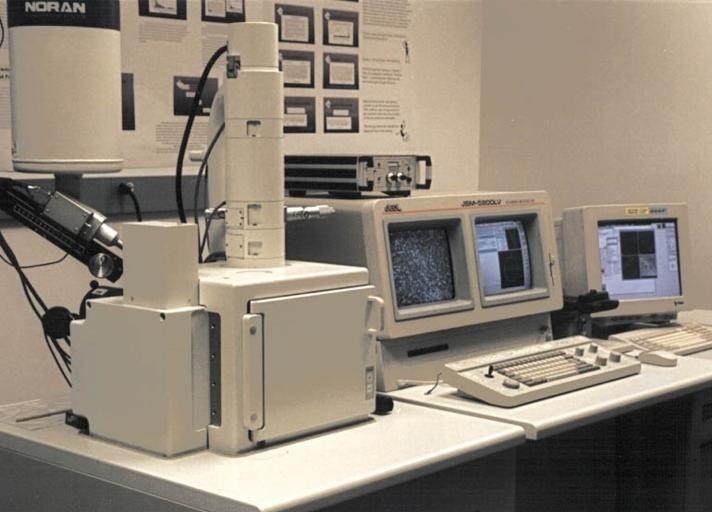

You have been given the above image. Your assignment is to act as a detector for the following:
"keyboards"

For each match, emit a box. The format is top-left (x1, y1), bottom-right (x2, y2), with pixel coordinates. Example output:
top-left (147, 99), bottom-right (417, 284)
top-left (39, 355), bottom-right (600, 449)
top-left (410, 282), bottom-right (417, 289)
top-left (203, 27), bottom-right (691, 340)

top-left (630, 322), bottom-right (712, 355)
top-left (441, 334), bottom-right (640, 412)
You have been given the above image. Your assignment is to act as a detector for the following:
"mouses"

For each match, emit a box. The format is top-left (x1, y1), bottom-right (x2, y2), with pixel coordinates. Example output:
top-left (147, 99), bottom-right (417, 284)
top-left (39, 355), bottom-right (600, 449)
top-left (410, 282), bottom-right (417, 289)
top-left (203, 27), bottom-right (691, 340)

top-left (638, 349), bottom-right (677, 367)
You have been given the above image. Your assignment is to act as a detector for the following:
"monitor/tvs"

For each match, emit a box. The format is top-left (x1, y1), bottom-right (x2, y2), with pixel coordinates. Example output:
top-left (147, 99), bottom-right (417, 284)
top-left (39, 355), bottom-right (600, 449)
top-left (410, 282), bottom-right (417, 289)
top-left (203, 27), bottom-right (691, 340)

top-left (382, 213), bottom-right (474, 321)
top-left (554, 202), bottom-right (686, 328)
top-left (470, 208), bottom-right (551, 307)
